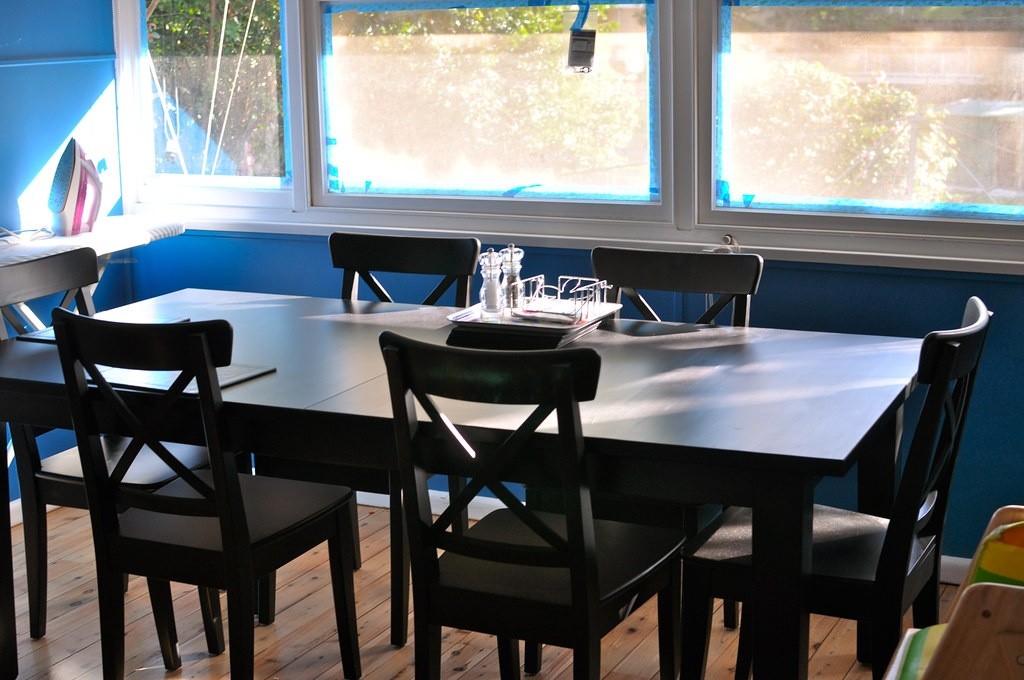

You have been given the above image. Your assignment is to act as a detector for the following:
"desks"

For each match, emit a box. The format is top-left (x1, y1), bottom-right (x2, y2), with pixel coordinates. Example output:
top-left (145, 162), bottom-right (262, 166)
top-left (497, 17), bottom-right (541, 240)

top-left (0, 288), bottom-right (926, 680)
top-left (0, 213), bottom-right (185, 343)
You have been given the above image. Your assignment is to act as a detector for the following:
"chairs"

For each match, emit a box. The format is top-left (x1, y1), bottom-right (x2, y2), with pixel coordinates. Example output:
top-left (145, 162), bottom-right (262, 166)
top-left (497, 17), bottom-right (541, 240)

top-left (376, 331), bottom-right (690, 680)
top-left (691, 295), bottom-right (996, 680)
top-left (253, 232), bottom-right (480, 653)
top-left (589, 245), bottom-right (765, 632)
top-left (0, 247), bottom-right (253, 671)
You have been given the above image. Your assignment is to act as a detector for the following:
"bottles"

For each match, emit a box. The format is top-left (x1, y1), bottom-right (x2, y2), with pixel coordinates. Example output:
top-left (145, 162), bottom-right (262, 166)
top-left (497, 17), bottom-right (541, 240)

top-left (500, 243), bottom-right (523, 312)
top-left (476, 248), bottom-right (505, 320)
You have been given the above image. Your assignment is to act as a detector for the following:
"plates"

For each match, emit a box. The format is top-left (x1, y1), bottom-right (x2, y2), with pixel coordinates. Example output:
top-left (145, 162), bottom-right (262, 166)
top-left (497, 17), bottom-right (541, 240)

top-left (447, 296), bottom-right (623, 349)
